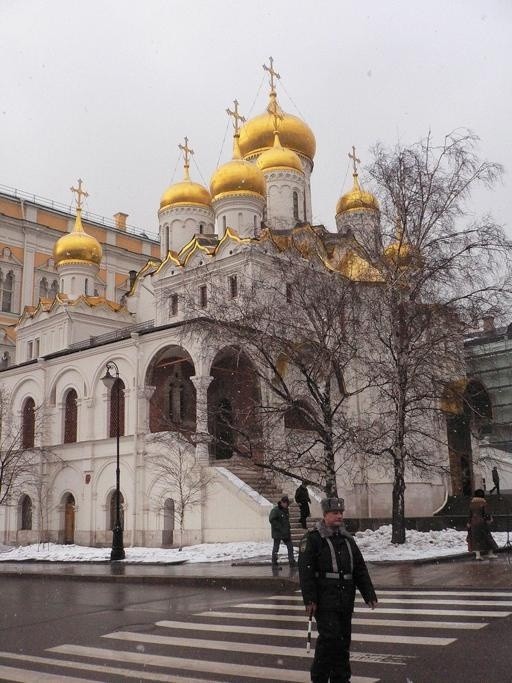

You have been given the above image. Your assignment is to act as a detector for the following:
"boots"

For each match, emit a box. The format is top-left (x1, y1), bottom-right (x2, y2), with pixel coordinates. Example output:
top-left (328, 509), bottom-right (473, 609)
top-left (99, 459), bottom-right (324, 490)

top-left (488, 550), bottom-right (498, 559)
top-left (475, 550), bottom-right (484, 560)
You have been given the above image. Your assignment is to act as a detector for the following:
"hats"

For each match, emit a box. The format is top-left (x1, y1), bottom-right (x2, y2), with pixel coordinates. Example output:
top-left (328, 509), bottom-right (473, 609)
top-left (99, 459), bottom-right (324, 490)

top-left (321, 497), bottom-right (345, 512)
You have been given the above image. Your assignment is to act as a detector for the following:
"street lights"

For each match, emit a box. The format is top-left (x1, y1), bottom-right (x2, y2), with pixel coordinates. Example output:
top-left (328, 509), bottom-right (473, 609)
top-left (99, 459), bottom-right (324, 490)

top-left (99, 360), bottom-right (124, 562)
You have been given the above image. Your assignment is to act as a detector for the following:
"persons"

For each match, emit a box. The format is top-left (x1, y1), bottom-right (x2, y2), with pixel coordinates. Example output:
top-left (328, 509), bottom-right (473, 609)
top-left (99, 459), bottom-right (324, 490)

top-left (296, 482), bottom-right (311, 528)
top-left (467, 489), bottom-right (498, 560)
top-left (490, 467), bottom-right (500, 494)
top-left (298, 499), bottom-right (377, 683)
top-left (269, 495), bottom-right (298, 570)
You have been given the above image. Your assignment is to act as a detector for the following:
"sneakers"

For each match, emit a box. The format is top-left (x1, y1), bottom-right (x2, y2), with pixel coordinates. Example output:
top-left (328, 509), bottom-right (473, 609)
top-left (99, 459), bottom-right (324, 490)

top-left (272, 566), bottom-right (283, 570)
top-left (290, 561), bottom-right (299, 568)
